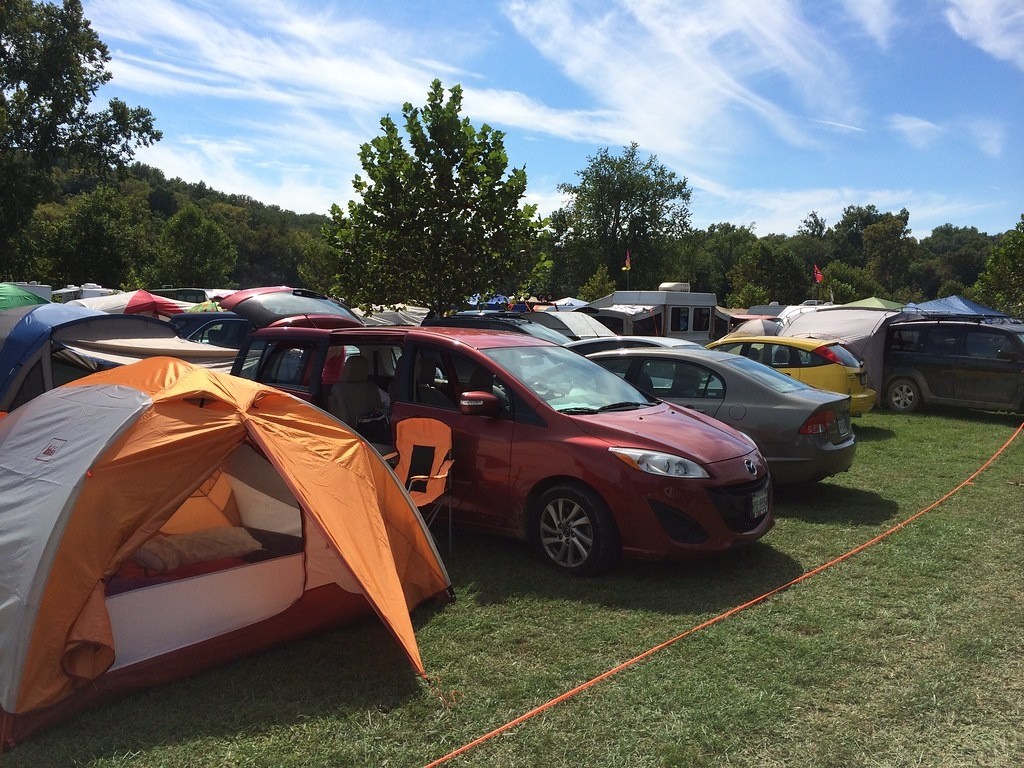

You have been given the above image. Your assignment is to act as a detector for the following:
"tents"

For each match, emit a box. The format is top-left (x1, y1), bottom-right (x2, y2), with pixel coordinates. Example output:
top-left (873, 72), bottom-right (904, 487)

top-left (730, 294), bottom-right (1009, 412)
top-left (354, 289), bottom-right (593, 327)
top-left (0, 282), bottom-right (226, 343)
top-left (0, 356), bottom-right (456, 751)
top-left (0, 303), bottom-right (304, 412)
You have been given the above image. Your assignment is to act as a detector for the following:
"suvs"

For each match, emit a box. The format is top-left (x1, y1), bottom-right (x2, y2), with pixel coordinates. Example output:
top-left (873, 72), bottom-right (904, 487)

top-left (880, 318), bottom-right (1024, 416)
top-left (224, 325), bottom-right (776, 580)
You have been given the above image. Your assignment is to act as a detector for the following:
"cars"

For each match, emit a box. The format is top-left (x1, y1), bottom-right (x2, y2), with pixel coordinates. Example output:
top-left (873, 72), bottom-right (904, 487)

top-left (135, 286), bottom-right (879, 499)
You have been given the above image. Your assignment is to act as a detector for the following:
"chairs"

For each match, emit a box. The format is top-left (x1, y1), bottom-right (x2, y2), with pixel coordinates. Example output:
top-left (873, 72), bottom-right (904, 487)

top-left (776, 351), bottom-right (790, 366)
top-left (207, 329), bottom-right (221, 344)
top-left (381, 417), bottom-right (456, 561)
top-left (668, 369), bottom-right (702, 397)
top-left (388, 356), bottom-right (456, 410)
top-left (325, 356), bottom-right (382, 429)
top-left (639, 373), bottom-right (654, 395)
top-left (748, 347), bottom-right (761, 361)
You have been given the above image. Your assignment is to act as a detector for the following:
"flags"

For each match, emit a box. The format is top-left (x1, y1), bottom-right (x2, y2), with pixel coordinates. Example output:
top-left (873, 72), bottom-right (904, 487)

top-left (814, 264), bottom-right (822, 283)
top-left (621, 248), bottom-right (630, 270)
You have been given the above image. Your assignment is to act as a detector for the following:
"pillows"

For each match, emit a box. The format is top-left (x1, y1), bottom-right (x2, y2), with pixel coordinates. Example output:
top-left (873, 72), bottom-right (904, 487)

top-left (132, 524), bottom-right (265, 577)
